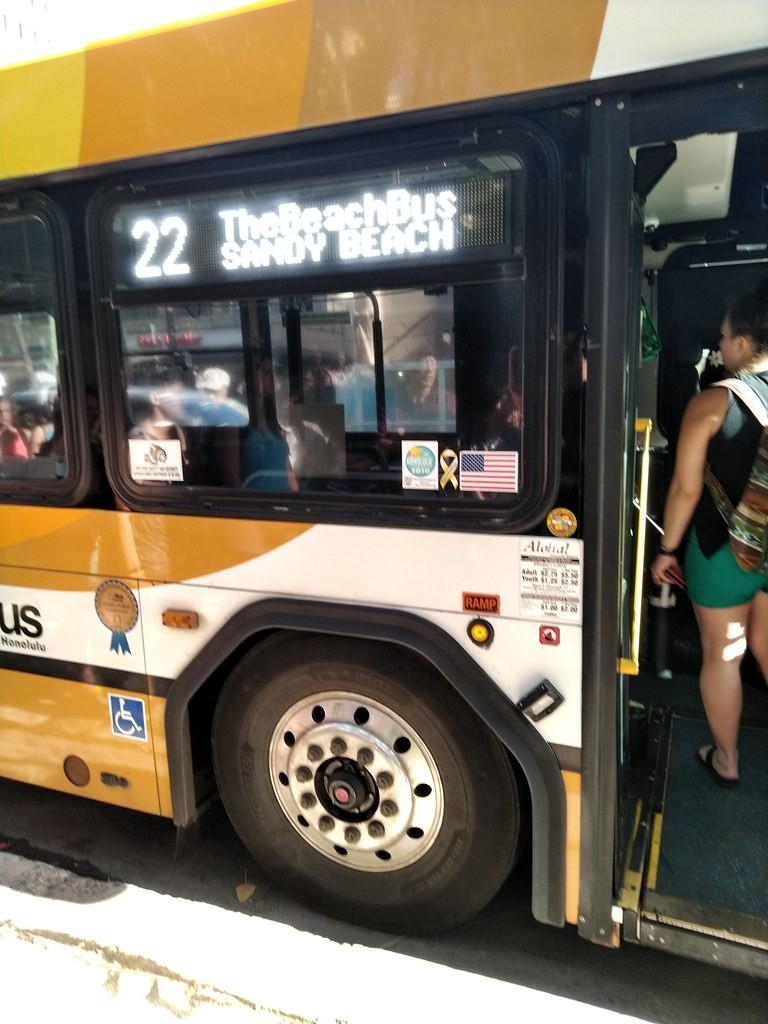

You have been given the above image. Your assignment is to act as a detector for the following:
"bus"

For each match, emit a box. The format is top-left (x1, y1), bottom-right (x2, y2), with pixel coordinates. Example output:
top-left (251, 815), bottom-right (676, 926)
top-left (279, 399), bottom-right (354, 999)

top-left (1, 0), bottom-right (768, 985)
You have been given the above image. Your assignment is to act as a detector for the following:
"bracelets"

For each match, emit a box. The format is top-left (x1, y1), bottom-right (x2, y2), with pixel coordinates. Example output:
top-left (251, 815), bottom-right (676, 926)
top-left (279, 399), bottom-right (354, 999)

top-left (658, 544), bottom-right (677, 555)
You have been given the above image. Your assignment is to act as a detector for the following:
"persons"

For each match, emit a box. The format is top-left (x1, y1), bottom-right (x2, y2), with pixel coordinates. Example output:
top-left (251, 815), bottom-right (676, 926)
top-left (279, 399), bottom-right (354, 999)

top-left (386, 345), bottom-right (456, 433)
top-left (198, 366), bottom-right (251, 426)
top-left (0, 391), bottom-right (65, 479)
top-left (234, 338), bottom-right (361, 493)
top-left (651, 299), bottom-right (768, 789)
top-left (126, 361), bottom-right (215, 486)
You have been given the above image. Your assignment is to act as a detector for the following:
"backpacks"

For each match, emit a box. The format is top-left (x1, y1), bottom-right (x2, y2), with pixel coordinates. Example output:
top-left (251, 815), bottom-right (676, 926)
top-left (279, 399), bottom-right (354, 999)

top-left (702, 377), bottom-right (768, 575)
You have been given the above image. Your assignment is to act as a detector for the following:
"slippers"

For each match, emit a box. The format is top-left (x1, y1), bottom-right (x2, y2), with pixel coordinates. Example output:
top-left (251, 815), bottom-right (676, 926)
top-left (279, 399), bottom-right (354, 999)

top-left (696, 744), bottom-right (739, 790)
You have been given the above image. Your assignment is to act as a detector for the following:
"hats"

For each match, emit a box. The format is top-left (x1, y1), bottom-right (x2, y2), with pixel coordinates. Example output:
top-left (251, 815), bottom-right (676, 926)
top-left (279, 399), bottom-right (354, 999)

top-left (196, 367), bottom-right (231, 391)
